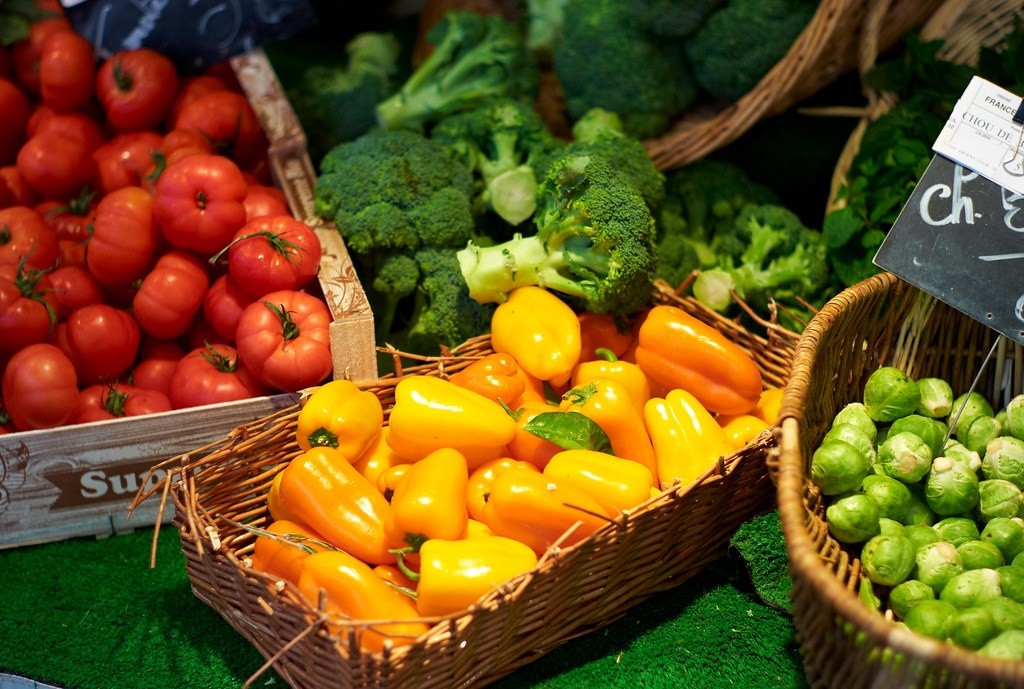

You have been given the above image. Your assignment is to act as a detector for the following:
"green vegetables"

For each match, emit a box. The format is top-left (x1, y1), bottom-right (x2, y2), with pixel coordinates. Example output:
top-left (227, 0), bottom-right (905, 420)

top-left (824, 30), bottom-right (1024, 289)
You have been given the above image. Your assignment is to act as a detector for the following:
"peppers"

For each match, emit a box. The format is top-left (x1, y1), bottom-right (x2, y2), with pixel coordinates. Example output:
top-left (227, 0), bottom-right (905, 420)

top-left (255, 283), bottom-right (782, 651)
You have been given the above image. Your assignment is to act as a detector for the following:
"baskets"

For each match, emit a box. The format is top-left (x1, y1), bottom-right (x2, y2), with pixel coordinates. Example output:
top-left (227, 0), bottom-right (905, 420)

top-left (127, 270), bottom-right (819, 689)
top-left (412, 0), bottom-right (1024, 287)
top-left (766, 271), bottom-right (1024, 689)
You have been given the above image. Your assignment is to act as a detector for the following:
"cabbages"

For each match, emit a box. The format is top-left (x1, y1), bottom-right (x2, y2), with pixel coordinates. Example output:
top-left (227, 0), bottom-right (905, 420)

top-left (812, 368), bottom-right (1024, 660)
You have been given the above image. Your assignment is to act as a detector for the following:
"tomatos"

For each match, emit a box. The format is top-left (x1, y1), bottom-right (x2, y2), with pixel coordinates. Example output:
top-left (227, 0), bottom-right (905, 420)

top-left (0, 0), bottom-right (333, 434)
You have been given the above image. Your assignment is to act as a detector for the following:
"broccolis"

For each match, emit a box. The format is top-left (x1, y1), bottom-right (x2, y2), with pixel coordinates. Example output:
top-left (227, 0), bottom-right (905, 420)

top-left (291, 0), bottom-right (832, 351)
